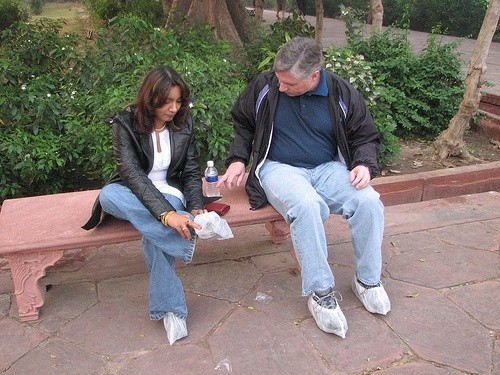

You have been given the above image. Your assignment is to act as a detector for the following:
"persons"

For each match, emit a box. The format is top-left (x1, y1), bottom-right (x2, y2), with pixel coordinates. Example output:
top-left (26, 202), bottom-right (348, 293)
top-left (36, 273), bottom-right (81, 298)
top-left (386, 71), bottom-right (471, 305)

top-left (81, 65), bottom-right (208, 345)
top-left (216, 36), bottom-right (391, 338)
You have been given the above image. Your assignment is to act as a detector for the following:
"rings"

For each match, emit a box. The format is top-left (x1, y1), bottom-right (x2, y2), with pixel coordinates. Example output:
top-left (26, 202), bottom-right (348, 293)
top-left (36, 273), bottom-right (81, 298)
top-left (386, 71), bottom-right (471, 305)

top-left (186, 220), bottom-right (191, 224)
top-left (181, 228), bottom-right (188, 231)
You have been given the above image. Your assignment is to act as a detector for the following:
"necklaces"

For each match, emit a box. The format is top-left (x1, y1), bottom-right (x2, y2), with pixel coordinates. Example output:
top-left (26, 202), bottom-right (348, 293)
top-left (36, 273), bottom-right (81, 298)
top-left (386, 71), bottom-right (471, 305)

top-left (150, 123), bottom-right (166, 132)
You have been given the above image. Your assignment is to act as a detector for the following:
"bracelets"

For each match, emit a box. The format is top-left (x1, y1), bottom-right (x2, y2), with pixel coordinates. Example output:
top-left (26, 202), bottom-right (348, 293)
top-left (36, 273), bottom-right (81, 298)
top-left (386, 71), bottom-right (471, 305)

top-left (159, 210), bottom-right (175, 227)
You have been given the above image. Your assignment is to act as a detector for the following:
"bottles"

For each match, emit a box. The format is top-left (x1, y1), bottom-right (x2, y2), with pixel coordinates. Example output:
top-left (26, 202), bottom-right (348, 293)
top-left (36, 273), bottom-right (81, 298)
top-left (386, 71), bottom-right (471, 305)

top-left (205, 161), bottom-right (218, 196)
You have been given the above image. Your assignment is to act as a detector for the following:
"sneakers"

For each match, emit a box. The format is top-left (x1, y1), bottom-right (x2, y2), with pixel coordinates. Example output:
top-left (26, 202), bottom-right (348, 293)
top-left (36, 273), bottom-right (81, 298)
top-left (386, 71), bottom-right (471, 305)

top-left (193, 211), bottom-right (234, 241)
top-left (351, 271), bottom-right (391, 315)
top-left (307, 287), bottom-right (348, 334)
top-left (162, 310), bottom-right (190, 340)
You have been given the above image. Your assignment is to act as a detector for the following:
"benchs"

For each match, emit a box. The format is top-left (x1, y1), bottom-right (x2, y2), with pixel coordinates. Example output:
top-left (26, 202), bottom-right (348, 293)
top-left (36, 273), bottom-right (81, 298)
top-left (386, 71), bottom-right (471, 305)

top-left (0, 173), bottom-right (304, 322)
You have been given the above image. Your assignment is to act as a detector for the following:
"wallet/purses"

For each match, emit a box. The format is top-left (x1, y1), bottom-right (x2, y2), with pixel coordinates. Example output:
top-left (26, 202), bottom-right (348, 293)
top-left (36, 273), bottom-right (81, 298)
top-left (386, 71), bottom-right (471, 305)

top-left (203, 203), bottom-right (230, 215)
